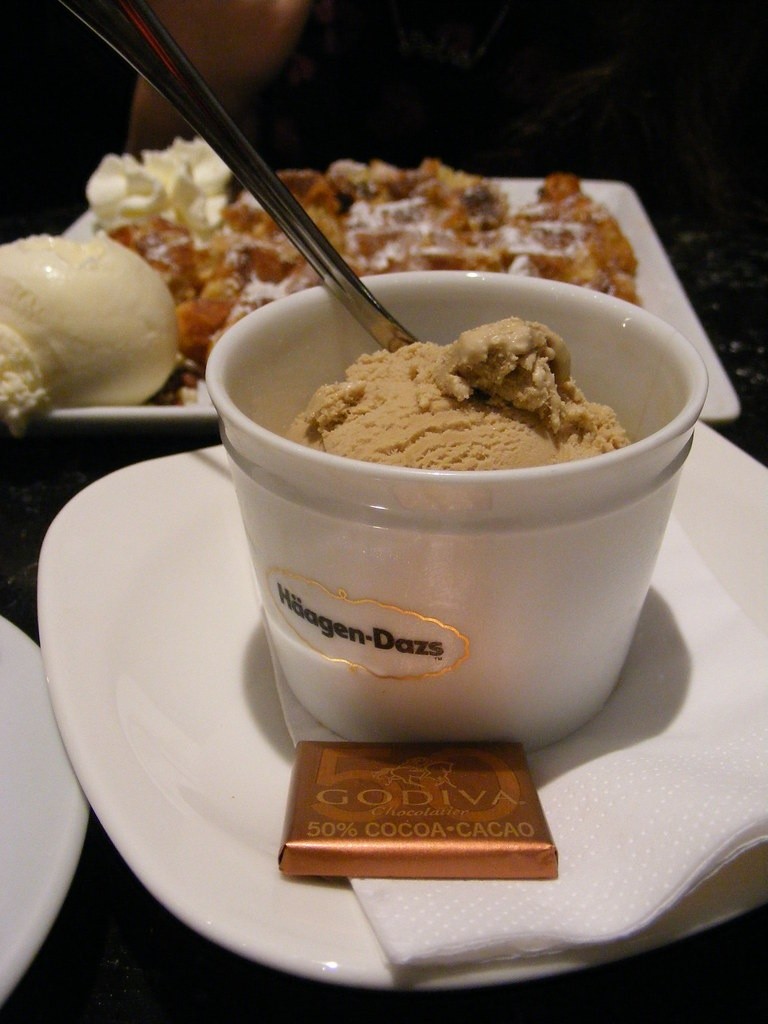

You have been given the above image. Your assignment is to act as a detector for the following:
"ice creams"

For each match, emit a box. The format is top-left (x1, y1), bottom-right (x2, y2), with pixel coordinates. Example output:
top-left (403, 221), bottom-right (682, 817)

top-left (287, 313), bottom-right (633, 472)
top-left (0, 232), bottom-right (180, 439)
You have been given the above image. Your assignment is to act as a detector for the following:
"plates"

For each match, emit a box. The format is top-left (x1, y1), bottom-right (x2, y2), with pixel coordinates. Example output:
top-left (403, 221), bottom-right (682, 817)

top-left (32, 421), bottom-right (768, 989)
top-left (0, 613), bottom-right (90, 1012)
top-left (44, 174), bottom-right (744, 433)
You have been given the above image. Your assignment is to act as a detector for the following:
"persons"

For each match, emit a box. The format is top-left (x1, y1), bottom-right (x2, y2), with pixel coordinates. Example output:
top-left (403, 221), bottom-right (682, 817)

top-left (122, 0), bottom-right (738, 200)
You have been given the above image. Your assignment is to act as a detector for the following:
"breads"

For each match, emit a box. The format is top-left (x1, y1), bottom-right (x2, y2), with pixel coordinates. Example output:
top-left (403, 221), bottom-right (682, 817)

top-left (104, 158), bottom-right (637, 373)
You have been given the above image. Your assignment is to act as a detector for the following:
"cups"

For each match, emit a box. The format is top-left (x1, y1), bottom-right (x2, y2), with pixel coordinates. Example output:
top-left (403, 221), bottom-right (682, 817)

top-left (201, 268), bottom-right (707, 754)
